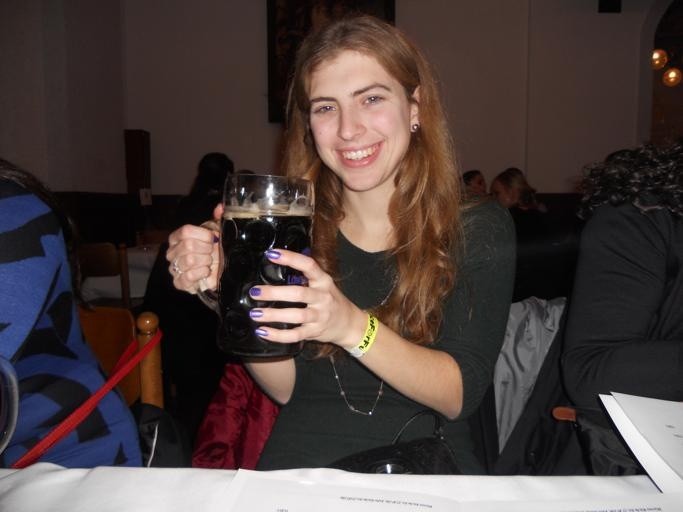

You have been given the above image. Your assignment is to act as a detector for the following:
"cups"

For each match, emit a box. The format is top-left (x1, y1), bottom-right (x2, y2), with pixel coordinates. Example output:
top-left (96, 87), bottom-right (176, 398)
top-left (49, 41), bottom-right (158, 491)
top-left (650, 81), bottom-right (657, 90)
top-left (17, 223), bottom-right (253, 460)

top-left (196, 174), bottom-right (316, 360)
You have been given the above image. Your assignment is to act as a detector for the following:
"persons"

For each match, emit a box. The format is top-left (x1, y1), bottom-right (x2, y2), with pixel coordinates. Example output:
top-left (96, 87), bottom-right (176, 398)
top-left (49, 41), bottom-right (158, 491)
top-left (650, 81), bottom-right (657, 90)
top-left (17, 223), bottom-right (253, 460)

top-left (502, 166), bottom-right (546, 212)
top-left (456, 169), bottom-right (488, 200)
top-left (184, 151), bottom-right (235, 196)
top-left (163, 13), bottom-right (520, 474)
top-left (492, 171), bottom-right (566, 304)
top-left (491, 133), bottom-right (683, 475)
top-left (230, 170), bottom-right (260, 203)
top-left (141, 189), bottom-right (233, 426)
top-left (1, 155), bottom-right (145, 468)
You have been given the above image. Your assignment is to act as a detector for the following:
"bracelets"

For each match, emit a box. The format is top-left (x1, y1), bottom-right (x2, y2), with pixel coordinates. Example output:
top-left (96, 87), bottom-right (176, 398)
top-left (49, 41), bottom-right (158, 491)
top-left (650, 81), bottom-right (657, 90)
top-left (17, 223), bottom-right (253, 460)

top-left (346, 311), bottom-right (378, 360)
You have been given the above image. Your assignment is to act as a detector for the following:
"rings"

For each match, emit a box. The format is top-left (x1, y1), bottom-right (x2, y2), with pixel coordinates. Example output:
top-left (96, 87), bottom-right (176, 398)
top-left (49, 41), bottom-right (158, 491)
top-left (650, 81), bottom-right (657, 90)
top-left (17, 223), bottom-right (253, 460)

top-left (172, 258), bottom-right (183, 275)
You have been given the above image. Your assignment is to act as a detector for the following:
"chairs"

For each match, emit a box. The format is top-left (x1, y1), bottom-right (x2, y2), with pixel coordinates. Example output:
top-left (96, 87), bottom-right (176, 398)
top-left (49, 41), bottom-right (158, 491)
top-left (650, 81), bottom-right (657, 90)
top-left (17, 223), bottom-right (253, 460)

top-left (76, 304), bottom-right (139, 408)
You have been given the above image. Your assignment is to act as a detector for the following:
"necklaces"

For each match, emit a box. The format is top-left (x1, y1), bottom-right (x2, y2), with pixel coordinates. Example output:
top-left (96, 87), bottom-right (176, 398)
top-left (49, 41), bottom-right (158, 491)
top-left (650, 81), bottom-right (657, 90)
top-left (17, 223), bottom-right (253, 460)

top-left (328, 268), bottom-right (399, 416)
